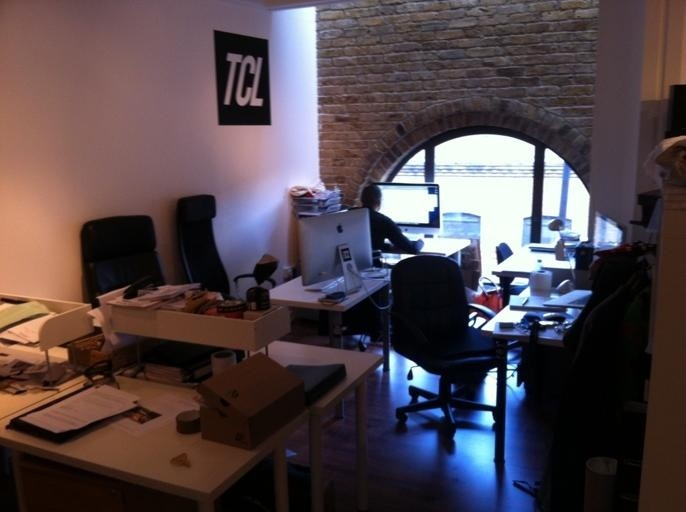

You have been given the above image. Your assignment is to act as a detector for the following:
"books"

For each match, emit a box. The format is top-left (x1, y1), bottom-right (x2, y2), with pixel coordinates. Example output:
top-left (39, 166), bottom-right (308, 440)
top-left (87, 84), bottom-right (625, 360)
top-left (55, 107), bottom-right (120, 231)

top-left (287, 362), bottom-right (346, 405)
top-left (106, 288), bottom-right (185, 311)
top-left (7, 382), bottom-right (141, 445)
top-left (509, 294), bottom-right (565, 311)
top-left (494, 320), bottom-right (563, 343)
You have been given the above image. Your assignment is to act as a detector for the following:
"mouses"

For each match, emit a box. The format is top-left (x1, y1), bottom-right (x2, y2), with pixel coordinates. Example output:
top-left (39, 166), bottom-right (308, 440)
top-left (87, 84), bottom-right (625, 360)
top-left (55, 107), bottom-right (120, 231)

top-left (522, 312), bottom-right (541, 326)
top-left (543, 313), bottom-right (566, 323)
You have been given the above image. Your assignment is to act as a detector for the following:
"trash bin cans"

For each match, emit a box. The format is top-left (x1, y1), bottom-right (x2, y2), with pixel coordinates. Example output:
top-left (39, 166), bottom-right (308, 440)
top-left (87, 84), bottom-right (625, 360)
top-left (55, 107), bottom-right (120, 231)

top-left (584, 457), bottom-right (618, 512)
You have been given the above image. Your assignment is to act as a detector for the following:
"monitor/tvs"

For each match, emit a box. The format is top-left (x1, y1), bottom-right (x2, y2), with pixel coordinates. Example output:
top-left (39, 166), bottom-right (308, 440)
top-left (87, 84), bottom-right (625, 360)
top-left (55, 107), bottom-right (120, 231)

top-left (297, 208), bottom-right (374, 296)
top-left (374, 182), bottom-right (441, 234)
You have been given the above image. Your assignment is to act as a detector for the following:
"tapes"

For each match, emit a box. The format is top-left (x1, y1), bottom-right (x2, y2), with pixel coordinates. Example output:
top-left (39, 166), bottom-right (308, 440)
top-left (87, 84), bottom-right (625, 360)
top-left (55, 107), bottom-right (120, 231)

top-left (211, 351), bottom-right (237, 378)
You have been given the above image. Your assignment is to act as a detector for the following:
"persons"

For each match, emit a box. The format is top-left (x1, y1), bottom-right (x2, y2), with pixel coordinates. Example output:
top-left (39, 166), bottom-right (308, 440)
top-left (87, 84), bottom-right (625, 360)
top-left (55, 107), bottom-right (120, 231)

top-left (350, 184), bottom-right (426, 269)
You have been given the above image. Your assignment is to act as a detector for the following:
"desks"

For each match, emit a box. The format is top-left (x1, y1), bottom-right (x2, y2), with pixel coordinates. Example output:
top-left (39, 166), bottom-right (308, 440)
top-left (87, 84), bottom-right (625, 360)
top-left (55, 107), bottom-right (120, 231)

top-left (481, 243), bottom-right (594, 464)
top-left (270, 267), bottom-right (392, 371)
top-left (382, 235), bottom-right (471, 267)
top-left (1, 341), bottom-right (383, 512)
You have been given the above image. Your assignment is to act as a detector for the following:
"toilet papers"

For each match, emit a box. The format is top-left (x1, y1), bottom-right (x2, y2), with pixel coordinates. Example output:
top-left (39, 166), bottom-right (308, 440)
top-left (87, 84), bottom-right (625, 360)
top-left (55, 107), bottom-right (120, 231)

top-left (529, 269), bottom-right (552, 299)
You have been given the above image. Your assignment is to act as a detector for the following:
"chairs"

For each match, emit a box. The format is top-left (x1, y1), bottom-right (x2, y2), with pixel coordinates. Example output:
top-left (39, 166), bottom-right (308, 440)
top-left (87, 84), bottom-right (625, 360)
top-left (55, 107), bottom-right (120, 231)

top-left (496, 242), bottom-right (530, 295)
top-left (80, 194), bottom-right (276, 308)
top-left (391, 255), bottom-right (508, 437)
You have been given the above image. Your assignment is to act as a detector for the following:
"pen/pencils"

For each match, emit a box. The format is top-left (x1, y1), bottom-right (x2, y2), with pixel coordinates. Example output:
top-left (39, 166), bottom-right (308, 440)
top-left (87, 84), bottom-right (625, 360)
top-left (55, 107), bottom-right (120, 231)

top-left (522, 298), bottom-right (528, 305)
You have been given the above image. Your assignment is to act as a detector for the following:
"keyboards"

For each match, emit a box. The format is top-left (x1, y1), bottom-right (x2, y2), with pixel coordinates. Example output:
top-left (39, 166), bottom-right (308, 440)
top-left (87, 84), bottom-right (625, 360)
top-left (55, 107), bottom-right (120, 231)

top-left (304, 276), bottom-right (343, 291)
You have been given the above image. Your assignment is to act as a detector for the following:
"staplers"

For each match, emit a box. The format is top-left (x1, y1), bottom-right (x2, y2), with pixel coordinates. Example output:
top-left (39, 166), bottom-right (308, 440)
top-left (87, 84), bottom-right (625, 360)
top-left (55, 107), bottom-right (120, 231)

top-left (123, 276), bottom-right (158, 299)
